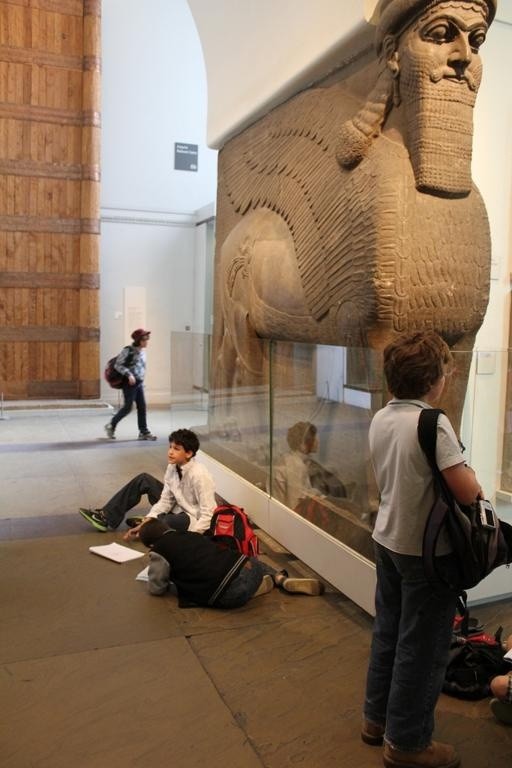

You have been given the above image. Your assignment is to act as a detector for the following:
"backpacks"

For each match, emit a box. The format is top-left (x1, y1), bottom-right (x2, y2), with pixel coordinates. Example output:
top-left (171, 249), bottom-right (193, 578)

top-left (203, 504), bottom-right (259, 558)
top-left (105, 346), bottom-right (133, 389)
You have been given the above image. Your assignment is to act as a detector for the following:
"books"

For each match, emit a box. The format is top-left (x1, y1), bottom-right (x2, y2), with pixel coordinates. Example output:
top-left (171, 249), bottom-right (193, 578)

top-left (502, 649), bottom-right (512, 663)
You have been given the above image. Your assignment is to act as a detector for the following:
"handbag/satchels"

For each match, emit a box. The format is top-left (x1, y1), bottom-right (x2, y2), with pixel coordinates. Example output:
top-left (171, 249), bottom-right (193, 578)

top-left (441, 592), bottom-right (511, 701)
top-left (417, 409), bottom-right (509, 598)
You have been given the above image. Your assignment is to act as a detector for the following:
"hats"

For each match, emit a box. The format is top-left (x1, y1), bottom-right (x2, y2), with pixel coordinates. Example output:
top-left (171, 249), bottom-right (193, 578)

top-left (132, 329), bottom-right (151, 340)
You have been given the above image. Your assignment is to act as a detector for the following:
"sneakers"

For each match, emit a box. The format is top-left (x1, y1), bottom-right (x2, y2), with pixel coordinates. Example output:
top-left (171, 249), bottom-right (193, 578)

top-left (381, 739), bottom-right (460, 767)
top-left (489, 698), bottom-right (511, 725)
top-left (251, 574), bottom-right (275, 598)
top-left (125, 516), bottom-right (146, 529)
top-left (105, 423), bottom-right (116, 439)
top-left (360, 714), bottom-right (384, 746)
top-left (139, 432), bottom-right (157, 441)
top-left (282, 577), bottom-right (325, 597)
top-left (79, 508), bottom-right (109, 533)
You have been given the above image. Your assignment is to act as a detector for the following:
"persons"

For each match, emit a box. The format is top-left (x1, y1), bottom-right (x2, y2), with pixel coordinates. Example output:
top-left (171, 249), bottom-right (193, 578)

top-left (104, 328), bottom-right (158, 441)
top-left (139, 518), bottom-right (324, 609)
top-left (264, 418), bottom-right (368, 522)
top-left (356, 328), bottom-right (486, 768)
top-left (488, 632), bottom-right (511, 728)
top-left (77, 428), bottom-right (220, 541)
